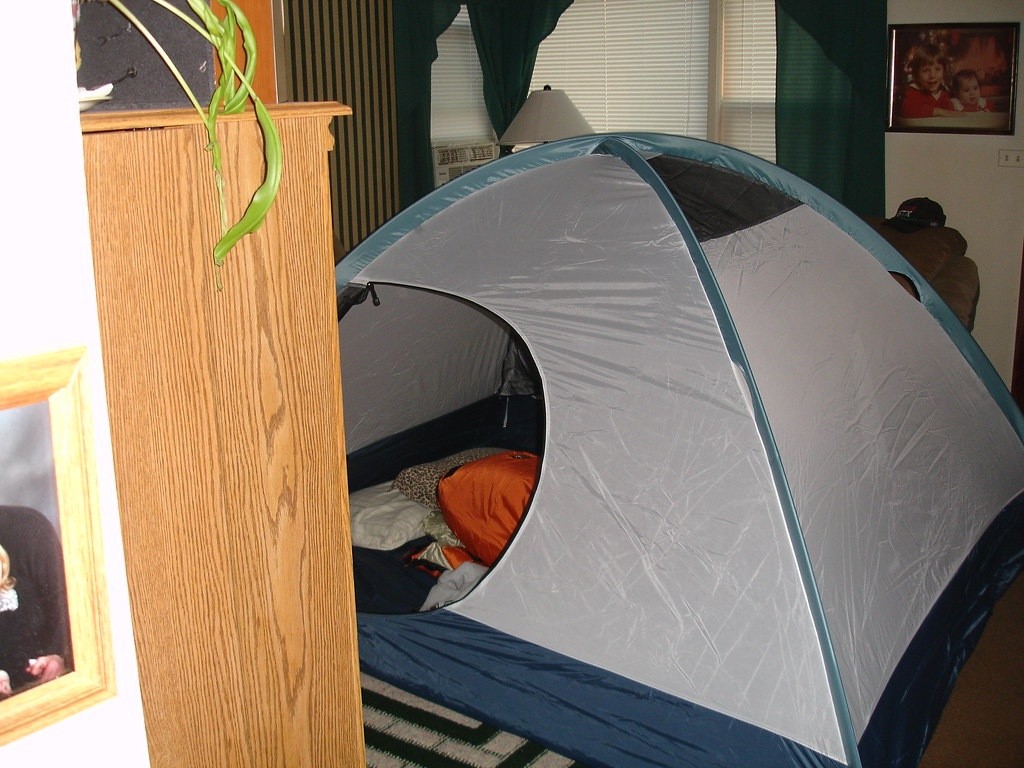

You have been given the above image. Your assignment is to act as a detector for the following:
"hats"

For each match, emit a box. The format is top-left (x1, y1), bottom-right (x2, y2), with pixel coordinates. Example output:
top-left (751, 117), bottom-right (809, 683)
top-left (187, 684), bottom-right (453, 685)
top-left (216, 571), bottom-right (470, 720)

top-left (882, 197), bottom-right (947, 233)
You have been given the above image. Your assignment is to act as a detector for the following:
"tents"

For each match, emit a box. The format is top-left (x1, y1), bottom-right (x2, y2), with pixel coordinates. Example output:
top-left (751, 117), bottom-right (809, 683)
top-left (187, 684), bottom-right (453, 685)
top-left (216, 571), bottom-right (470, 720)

top-left (334, 131), bottom-right (1024, 768)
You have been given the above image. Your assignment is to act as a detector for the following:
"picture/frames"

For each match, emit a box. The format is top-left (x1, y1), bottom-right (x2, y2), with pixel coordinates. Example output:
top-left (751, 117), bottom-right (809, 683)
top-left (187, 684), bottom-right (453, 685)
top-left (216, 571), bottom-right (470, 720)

top-left (0, 344), bottom-right (120, 746)
top-left (884, 22), bottom-right (1020, 136)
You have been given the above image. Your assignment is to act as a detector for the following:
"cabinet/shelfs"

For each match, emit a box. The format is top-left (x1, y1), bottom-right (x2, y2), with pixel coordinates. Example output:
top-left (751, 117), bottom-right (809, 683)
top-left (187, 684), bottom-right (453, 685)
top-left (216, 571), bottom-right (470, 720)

top-left (81, 100), bottom-right (368, 768)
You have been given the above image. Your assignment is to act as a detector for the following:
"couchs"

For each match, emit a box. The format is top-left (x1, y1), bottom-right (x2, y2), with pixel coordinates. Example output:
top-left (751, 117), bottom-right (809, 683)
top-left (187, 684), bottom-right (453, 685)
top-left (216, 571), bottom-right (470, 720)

top-left (861, 215), bottom-right (980, 333)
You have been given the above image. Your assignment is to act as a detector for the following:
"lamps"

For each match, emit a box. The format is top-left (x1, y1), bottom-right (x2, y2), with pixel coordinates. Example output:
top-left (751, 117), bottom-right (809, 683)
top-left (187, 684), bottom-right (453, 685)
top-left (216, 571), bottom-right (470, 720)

top-left (496, 86), bottom-right (595, 144)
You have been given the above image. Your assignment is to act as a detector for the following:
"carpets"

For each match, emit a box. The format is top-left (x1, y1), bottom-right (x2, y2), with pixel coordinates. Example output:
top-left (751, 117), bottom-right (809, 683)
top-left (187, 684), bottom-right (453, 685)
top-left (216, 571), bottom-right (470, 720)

top-left (360, 673), bottom-right (588, 768)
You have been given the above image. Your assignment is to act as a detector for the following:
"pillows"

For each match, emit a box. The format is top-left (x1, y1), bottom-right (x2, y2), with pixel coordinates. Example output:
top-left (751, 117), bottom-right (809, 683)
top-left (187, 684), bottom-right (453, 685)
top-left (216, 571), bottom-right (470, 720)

top-left (349, 482), bottom-right (433, 552)
top-left (438, 450), bottom-right (541, 564)
top-left (397, 447), bottom-right (513, 509)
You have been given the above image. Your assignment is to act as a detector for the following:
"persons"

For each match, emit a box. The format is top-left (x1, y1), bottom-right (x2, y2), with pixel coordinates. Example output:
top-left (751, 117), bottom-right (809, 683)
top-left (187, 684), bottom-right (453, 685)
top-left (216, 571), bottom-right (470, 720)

top-left (946, 69), bottom-right (997, 112)
top-left (0, 546), bottom-right (65, 695)
top-left (901, 43), bottom-right (965, 117)
